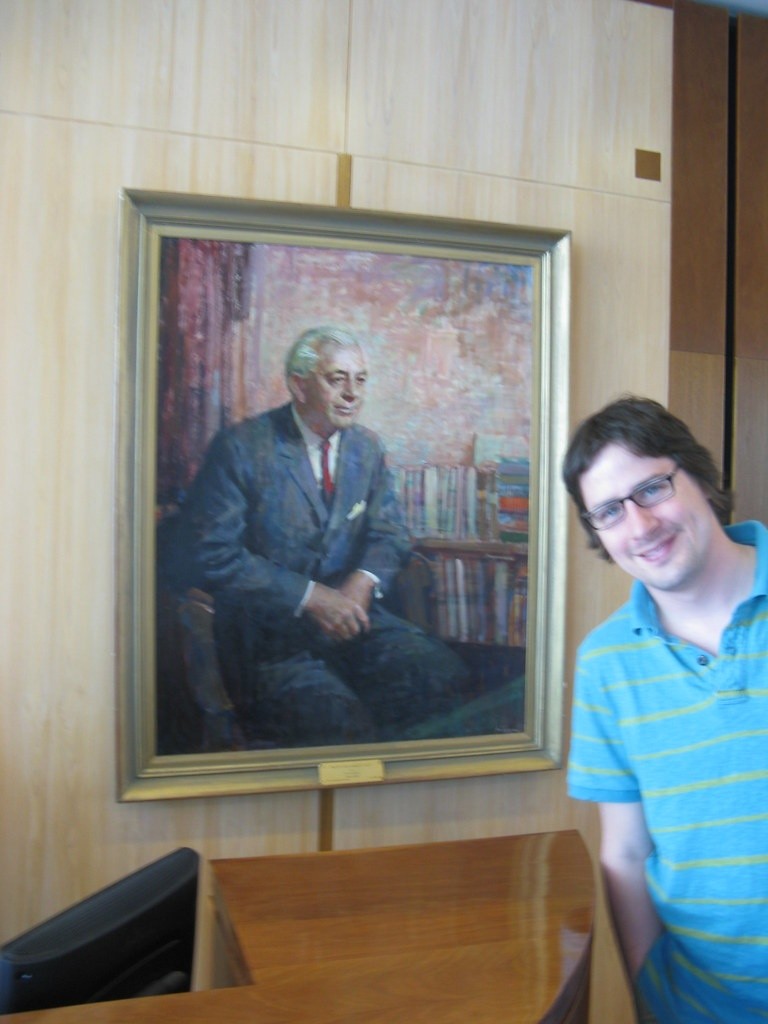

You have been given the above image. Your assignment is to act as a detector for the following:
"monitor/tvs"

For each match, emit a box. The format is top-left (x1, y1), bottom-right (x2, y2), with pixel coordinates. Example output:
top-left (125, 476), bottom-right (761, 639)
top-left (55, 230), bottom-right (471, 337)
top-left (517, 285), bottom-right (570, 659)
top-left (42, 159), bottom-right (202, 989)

top-left (0, 846), bottom-right (201, 1012)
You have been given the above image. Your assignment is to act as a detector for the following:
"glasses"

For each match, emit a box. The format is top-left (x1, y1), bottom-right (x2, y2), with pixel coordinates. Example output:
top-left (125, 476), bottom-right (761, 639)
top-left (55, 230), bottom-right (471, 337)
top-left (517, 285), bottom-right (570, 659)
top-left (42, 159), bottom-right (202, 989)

top-left (579, 462), bottom-right (681, 531)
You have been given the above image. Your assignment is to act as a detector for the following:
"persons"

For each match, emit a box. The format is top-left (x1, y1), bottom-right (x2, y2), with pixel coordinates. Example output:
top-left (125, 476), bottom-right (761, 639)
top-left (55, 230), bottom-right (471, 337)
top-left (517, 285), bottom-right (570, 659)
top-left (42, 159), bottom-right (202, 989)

top-left (562, 398), bottom-right (768, 1023)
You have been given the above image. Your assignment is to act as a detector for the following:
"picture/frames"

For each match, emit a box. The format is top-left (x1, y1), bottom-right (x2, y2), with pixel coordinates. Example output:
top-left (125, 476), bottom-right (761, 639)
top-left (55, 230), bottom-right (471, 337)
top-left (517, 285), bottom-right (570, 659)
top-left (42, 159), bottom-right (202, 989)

top-left (112, 185), bottom-right (576, 805)
